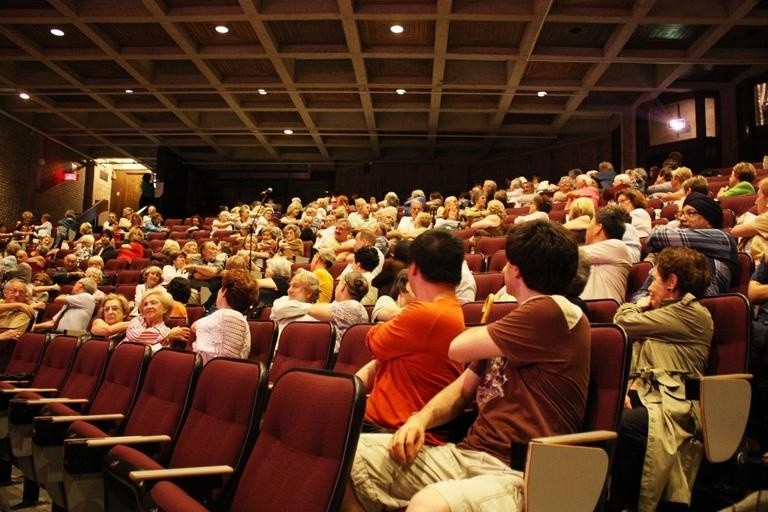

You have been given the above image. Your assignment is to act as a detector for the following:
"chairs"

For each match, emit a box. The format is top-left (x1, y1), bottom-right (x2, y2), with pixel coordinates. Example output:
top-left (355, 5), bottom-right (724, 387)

top-left (585, 297), bottom-right (622, 325)
top-left (57, 250), bottom-right (75, 258)
top-left (149, 239), bottom-right (165, 252)
top-left (438, 187), bottom-right (573, 235)
top-left (52, 258), bottom-right (66, 268)
top-left (146, 214), bottom-right (217, 239)
top-left (0, 334), bottom-right (78, 470)
top-left (302, 240), bottom-right (314, 259)
top-left (129, 368), bottom-right (367, 512)
top-left (98, 285), bottom-right (113, 294)
top-left (115, 270), bottom-right (143, 285)
top-left (627, 260), bottom-right (656, 300)
top-left (0, 333), bottom-right (51, 421)
top-left (10, 343), bottom-right (150, 509)
top-left (33, 347), bottom-right (203, 512)
top-left (184, 304), bottom-right (205, 324)
top-left (637, 159), bottom-right (768, 250)
top-left (465, 251), bottom-right (487, 274)
top-left (87, 357), bottom-right (268, 512)
top-left (223, 237), bottom-right (238, 249)
top-left (0, 338), bottom-right (113, 484)
top-left (481, 322), bottom-right (632, 512)
top-left (113, 284), bottom-right (138, 301)
top-left (470, 271), bottom-right (505, 304)
top-left (175, 240), bottom-right (189, 251)
top-left (463, 301), bottom-right (519, 326)
top-left (693, 291), bottom-right (753, 463)
top-left (197, 238), bottom-right (214, 251)
top-left (243, 320), bottom-right (279, 369)
top-left (486, 248), bottom-right (510, 273)
top-left (268, 321), bottom-right (336, 393)
top-left (103, 271), bottom-right (117, 284)
top-left (291, 262), bottom-right (312, 276)
top-left (39, 302), bottom-right (64, 329)
top-left (330, 262), bottom-right (346, 278)
top-left (105, 259), bottom-right (128, 271)
top-left (60, 283), bottom-right (73, 295)
top-left (333, 323), bottom-right (394, 373)
top-left (474, 235), bottom-right (508, 258)
top-left (168, 315), bottom-right (187, 351)
top-left (723, 251), bottom-right (753, 294)
top-left (131, 257), bottom-right (150, 270)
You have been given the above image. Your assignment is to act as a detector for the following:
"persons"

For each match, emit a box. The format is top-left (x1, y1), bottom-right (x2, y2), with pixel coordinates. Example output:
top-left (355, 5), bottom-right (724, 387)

top-left (267, 268), bottom-right (333, 376)
top-left (633, 192), bottom-right (732, 304)
top-left (30, 277), bottom-right (97, 347)
top-left (85, 267), bottom-right (108, 302)
top-left (185, 268), bottom-right (258, 383)
top-left (51, 202), bottom-right (163, 261)
top-left (351, 218), bottom-right (592, 511)
top-left (253, 257), bottom-right (293, 319)
top-left (159, 252), bottom-right (188, 291)
top-left (185, 214), bottom-right (204, 240)
top-left (603, 206), bottom-right (642, 265)
top-left (182, 241), bottom-right (223, 315)
top-left (0, 210), bottom-right (52, 284)
top-left (137, 171), bottom-right (159, 207)
top-left (504, 166), bottom-right (599, 230)
top-left (0, 301), bottom-right (41, 343)
top-left (87, 256), bottom-right (111, 285)
top-left (598, 158), bottom-right (625, 208)
top-left (731, 177), bottom-right (768, 271)
top-left (115, 290), bottom-right (191, 360)
top-left (211, 198), bottom-right (304, 268)
top-left (354, 228), bottom-right (466, 439)
top-left (0, 277), bottom-right (36, 343)
top-left (128, 265), bottom-right (173, 317)
top-left (351, 246), bottom-right (379, 306)
top-left (615, 188), bottom-right (651, 239)
top-left (455, 257), bottom-right (478, 304)
top-left (163, 226), bottom-right (179, 266)
top-left (26, 273), bottom-right (61, 317)
top-left (739, 251), bottom-right (768, 456)
top-left (85, 293), bottom-right (130, 345)
top-left (52, 254), bottom-right (85, 286)
top-left (574, 209), bottom-right (632, 308)
top-left (308, 272), bottom-right (369, 374)
top-left (716, 161), bottom-right (756, 200)
top-left (626, 149), bottom-right (714, 205)
top-left (310, 251), bottom-right (338, 305)
top-left (180, 240), bottom-right (202, 267)
top-left (371, 268), bottom-right (419, 322)
top-left (353, 232), bottom-right (384, 278)
top-left (612, 246), bottom-right (714, 512)
top-left (162, 277), bottom-right (191, 329)
top-left (374, 175), bottom-right (504, 262)
top-left (304, 196), bottom-right (334, 251)
top-left (334, 194), bottom-right (374, 231)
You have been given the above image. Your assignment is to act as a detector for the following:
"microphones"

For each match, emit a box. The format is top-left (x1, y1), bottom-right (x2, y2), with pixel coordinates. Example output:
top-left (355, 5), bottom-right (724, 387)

top-left (260, 188), bottom-right (272, 196)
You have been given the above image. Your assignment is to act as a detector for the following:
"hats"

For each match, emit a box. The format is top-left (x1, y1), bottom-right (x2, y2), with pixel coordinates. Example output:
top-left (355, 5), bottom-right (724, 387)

top-left (682, 193), bottom-right (723, 229)
top-left (3, 271), bottom-right (33, 281)
